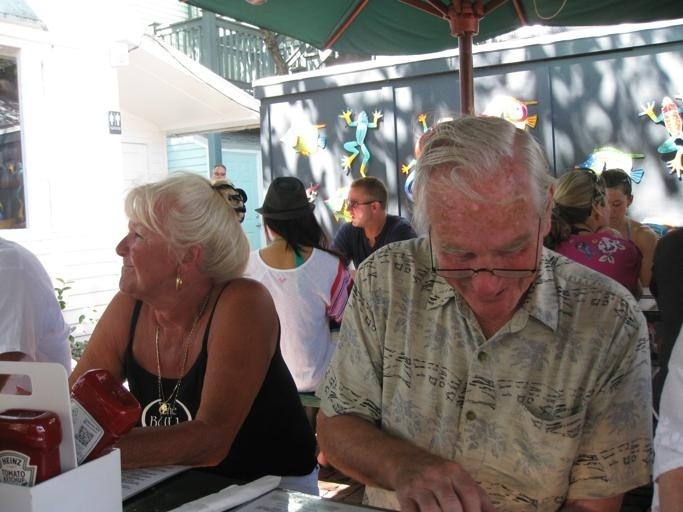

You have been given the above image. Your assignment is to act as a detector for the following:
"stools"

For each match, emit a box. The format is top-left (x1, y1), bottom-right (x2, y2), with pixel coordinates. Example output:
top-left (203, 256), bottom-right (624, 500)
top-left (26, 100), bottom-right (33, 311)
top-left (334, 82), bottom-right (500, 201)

top-left (296, 390), bottom-right (322, 429)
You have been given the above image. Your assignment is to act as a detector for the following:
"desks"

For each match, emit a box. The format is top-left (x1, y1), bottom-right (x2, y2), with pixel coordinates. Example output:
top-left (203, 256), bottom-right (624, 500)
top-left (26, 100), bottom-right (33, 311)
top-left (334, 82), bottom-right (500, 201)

top-left (626, 283), bottom-right (662, 424)
top-left (109, 458), bottom-right (397, 512)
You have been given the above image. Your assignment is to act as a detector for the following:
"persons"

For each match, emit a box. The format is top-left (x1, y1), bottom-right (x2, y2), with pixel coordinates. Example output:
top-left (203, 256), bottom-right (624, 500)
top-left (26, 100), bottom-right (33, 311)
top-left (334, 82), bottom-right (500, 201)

top-left (242, 172), bottom-right (356, 471)
top-left (12, 164), bottom-right (321, 512)
top-left (542, 162), bottom-right (643, 305)
top-left (0, 235), bottom-right (73, 413)
top-left (329, 175), bottom-right (416, 269)
top-left (648, 225), bottom-right (683, 438)
top-left (598, 166), bottom-right (661, 289)
top-left (211, 163), bottom-right (228, 180)
top-left (648, 320), bottom-right (683, 512)
top-left (311, 110), bottom-right (657, 512)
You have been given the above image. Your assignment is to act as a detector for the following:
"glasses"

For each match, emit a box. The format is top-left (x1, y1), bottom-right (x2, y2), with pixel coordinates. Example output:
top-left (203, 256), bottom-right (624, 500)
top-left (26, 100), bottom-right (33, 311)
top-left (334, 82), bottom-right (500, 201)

top-left (602, 171), bottom-right (631, 194)
top-left (429, 217), bottom-right (541, 280)
top-left (576, 168), bottom-right (600, 216)
top-left (347, 200), bottom-right (382, 208)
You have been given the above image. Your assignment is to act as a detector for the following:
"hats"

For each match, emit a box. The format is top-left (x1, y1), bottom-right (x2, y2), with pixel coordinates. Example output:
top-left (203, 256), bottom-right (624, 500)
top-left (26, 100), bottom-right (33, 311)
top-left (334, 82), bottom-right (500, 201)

top-left (255, 177), bottom-right (315, 219)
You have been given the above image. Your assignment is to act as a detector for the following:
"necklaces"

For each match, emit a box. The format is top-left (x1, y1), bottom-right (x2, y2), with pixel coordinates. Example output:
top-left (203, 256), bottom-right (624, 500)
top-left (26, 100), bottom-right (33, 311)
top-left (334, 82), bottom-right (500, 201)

top-left (154, 281), bottom-right (215, 418)
